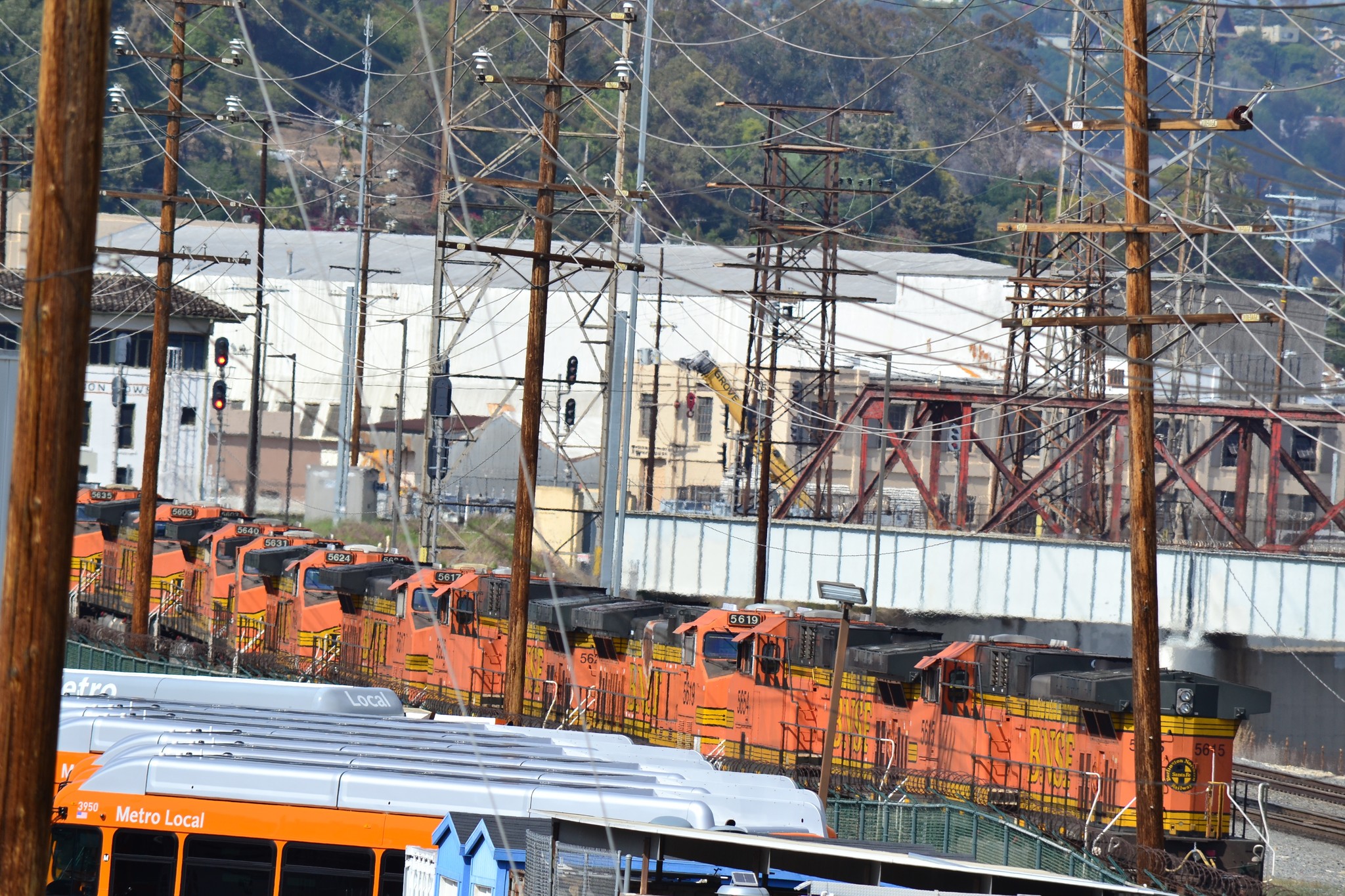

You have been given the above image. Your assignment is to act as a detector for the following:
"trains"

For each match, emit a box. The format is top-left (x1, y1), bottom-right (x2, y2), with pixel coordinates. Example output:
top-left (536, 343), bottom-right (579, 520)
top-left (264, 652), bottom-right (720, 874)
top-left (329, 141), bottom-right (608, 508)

top-left (40, 672), bottom-right (836, 896)
top-left (66, 484), bottom-right (1277, 883)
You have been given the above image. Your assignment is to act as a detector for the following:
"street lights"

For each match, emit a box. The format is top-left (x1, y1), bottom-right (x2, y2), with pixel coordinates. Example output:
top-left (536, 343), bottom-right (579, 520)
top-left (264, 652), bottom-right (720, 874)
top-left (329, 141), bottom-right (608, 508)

top-left (267, 353), bottom-right (296, 522)
top-left (376, 318), bottom-right (408, 552)
top-left (244, 303), bottom-right (271, 461)
top-left (854, 352), bottom-right (893, 624)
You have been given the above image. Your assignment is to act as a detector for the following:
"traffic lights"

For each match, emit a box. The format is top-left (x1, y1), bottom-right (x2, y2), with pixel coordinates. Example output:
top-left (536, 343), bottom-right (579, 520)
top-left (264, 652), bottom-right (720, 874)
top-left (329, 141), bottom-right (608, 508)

top-left (790, 415), bottom-right (801, 446)
top-left (211, 380), bottom-right (227, 412)
top-left (564, 355), bottom-right (579, 386)
top-left (563, 398), bottom-right (576, 429)
top-left (790, 381), bottom-right (802, 411)
top-left (214, 337), bottom-right (229, 368)
